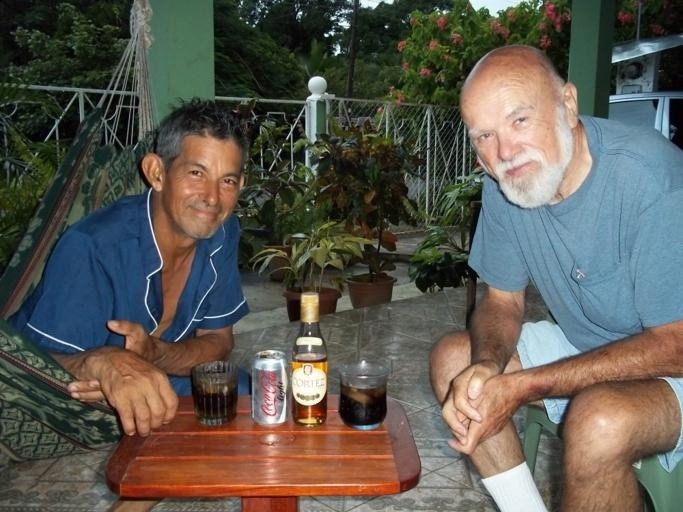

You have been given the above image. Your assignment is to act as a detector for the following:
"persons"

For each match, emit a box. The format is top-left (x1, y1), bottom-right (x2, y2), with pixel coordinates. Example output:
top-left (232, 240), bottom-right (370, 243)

top-left (0, 99), bottom-right (253, 473)
top-left (427, 42), bottom-right (683, 509)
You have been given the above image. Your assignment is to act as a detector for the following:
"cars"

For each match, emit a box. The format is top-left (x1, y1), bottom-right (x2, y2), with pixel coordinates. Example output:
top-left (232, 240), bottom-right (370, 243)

top-left (609, 90), bottom-right (682, 157)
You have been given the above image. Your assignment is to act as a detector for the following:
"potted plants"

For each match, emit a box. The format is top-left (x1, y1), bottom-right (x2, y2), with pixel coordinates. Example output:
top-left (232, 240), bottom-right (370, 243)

top-left (234, 121), bottom-right (424, 323)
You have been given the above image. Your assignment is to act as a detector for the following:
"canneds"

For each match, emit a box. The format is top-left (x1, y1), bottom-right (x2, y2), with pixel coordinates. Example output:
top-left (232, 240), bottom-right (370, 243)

top-left (251, 350), bottom-right (289, 428)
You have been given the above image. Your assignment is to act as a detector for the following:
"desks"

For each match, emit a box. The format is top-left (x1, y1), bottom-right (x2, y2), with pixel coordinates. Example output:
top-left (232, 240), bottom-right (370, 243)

top-left (104, 394), bottom-right (421, 512)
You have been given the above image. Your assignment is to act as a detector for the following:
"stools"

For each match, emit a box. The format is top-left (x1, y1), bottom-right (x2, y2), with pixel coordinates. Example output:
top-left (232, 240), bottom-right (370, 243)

top-left (522, 381), bottom-right (683, 511)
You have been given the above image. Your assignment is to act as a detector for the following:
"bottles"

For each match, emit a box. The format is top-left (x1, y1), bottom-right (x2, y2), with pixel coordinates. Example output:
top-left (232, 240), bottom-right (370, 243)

top-left (290, 291), bottom-right (329, 429)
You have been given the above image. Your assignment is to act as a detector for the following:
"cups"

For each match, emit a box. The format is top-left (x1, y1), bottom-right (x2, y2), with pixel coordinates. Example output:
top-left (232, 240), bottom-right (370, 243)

top-left (338, 361), bottom-right (390, 432)
top-left (189, 359), bottom-right (239, 427)
